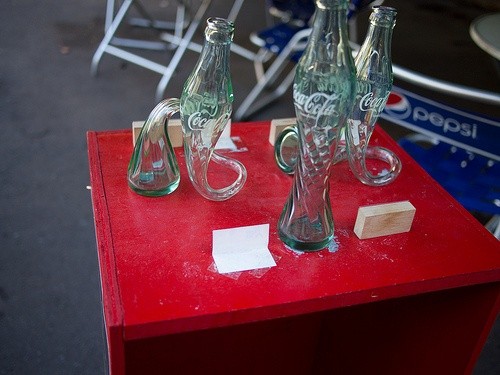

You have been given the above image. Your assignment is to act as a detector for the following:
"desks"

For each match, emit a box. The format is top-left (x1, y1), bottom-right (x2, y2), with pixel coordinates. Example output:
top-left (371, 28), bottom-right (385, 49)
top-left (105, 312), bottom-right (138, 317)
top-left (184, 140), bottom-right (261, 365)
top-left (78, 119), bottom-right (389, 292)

top-left (88, 118), bottom-right (500, 375)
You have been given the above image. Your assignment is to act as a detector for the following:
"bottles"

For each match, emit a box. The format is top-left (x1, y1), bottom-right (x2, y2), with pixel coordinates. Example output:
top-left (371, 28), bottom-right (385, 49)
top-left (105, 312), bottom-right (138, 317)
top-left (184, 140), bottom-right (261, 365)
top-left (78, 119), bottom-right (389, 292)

top-left (124, 15), bottom-right (249, 203)
top-left (274, 0), bottom-right (359, 253)
top-left (273, 4), bottom-right (402, 187)
